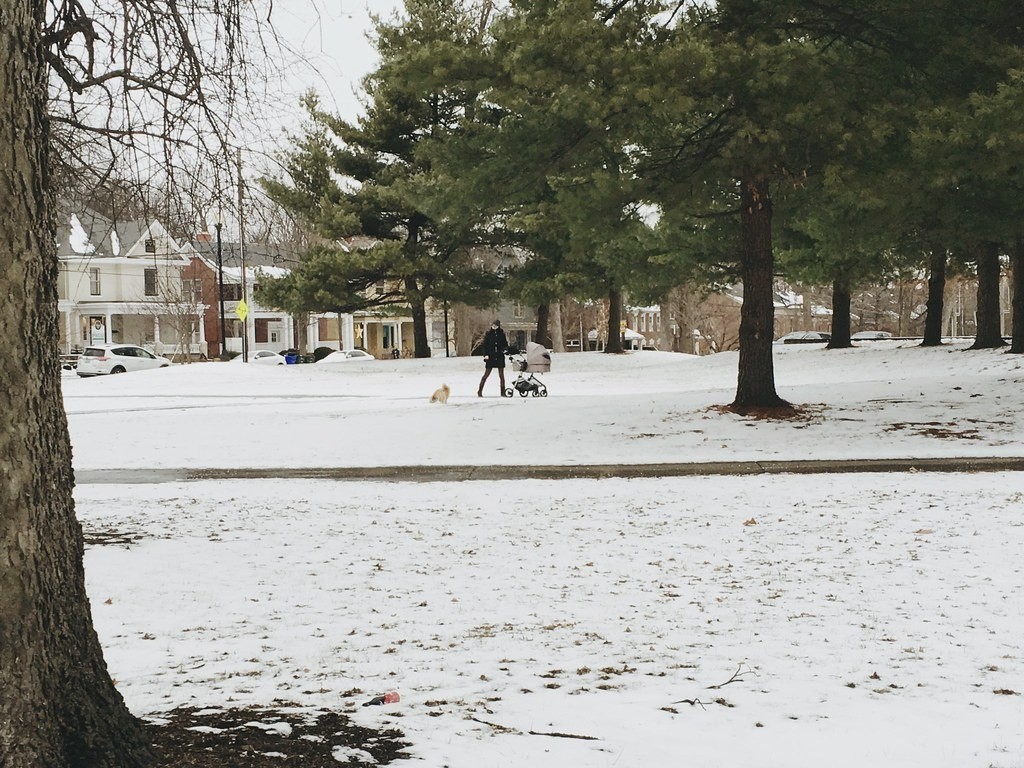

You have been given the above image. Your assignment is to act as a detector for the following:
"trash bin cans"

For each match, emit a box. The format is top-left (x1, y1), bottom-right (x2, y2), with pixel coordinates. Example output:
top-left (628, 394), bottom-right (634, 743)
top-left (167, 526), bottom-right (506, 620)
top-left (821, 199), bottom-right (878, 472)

top-left (284, 356), bottom-right (298, 364)
top-left (302, 354), bottom-right (315, 364)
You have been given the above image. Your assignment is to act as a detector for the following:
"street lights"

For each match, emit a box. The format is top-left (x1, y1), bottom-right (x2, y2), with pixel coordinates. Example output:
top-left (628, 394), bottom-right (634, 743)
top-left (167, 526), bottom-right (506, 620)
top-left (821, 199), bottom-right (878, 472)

top-left (214, 210), bottom-right (231, 361)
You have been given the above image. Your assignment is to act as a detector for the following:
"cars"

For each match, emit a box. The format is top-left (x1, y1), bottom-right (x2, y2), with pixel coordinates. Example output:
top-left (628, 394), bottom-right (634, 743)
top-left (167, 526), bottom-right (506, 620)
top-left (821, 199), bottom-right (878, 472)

top-left (229, 350), bottom-right (288, 366)
top-left (316, 350), bottom-right (375, 365)
top-left (776, 331), bottom-right (892, 343)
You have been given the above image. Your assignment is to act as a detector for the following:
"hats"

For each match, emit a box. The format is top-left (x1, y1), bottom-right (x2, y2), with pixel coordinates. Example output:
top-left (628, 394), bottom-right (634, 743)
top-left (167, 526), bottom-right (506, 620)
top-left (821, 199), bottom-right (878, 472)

top-left (491, 319), bottom-right (500, 326)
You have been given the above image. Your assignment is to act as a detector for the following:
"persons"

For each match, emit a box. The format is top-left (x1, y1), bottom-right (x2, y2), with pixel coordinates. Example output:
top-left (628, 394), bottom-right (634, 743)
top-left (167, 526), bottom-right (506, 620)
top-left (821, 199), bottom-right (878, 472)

top-left (478, 319), bottom-right (509, 397)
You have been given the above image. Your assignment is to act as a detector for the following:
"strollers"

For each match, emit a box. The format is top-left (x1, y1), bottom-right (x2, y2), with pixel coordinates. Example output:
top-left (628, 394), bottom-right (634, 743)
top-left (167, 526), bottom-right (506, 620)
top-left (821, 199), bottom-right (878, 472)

top-left (504, 342), bottom-right (552, 398)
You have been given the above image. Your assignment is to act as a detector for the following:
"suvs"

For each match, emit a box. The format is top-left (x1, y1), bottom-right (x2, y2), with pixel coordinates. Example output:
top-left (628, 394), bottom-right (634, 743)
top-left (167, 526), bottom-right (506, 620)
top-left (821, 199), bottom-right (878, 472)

top-left (76, 344), bottom-right (172, 380)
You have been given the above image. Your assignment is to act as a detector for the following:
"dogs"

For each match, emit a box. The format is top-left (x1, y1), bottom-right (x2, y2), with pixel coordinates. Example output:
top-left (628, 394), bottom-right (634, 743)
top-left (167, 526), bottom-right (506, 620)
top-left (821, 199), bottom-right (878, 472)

top-left (430, 383), bottom-right (450, 404)
